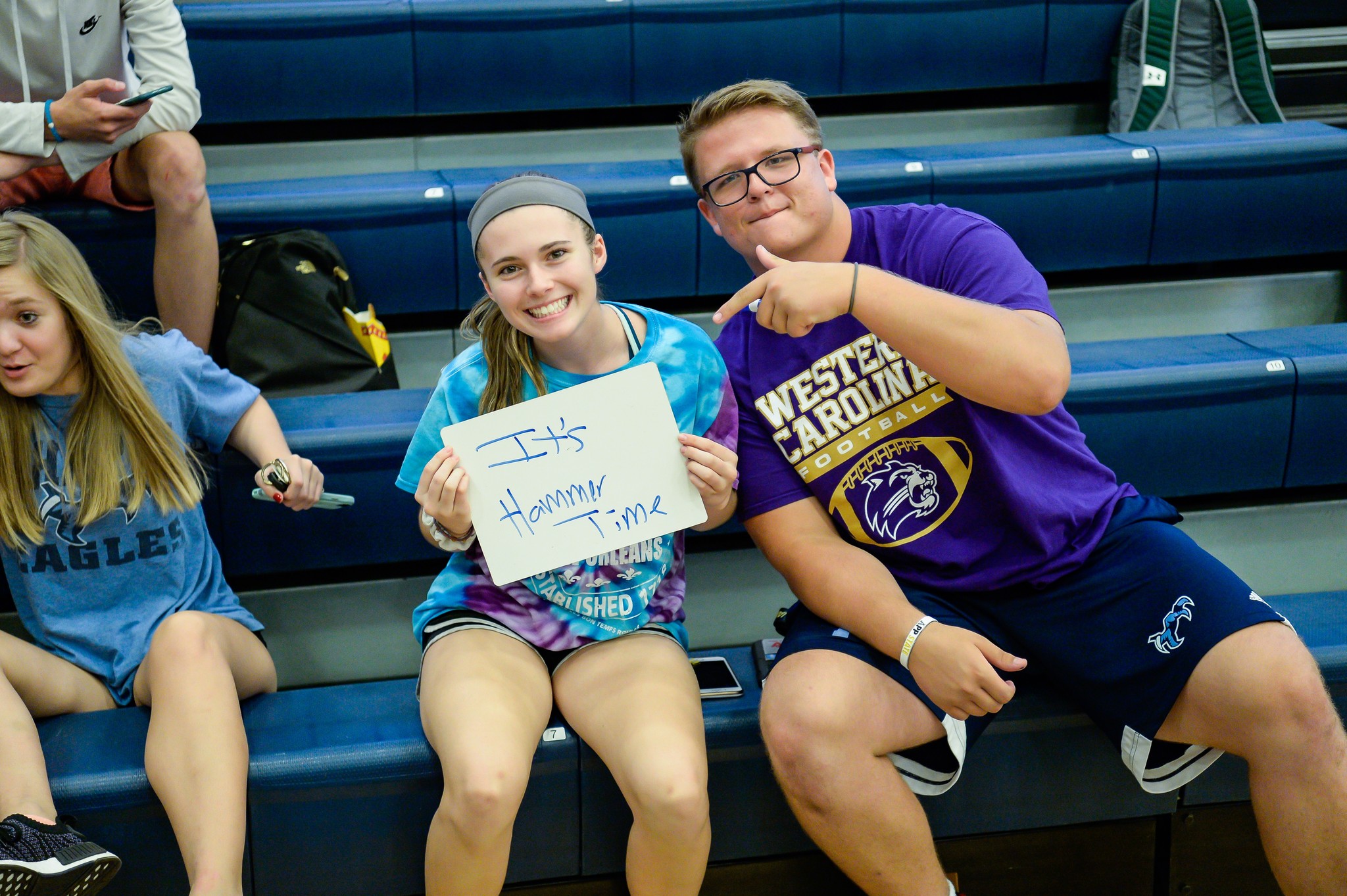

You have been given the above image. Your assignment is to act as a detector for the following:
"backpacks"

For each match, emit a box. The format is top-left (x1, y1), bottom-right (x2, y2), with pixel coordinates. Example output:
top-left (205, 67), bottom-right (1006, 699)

top-left (1105, 0), bottom-right (1288, 135)
top-left (209, 226), bottom-right (401, 396)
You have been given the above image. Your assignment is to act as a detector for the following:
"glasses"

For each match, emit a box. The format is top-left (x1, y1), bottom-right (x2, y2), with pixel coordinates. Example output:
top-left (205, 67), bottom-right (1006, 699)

top-left (701, 143), bottom-right (821, 207)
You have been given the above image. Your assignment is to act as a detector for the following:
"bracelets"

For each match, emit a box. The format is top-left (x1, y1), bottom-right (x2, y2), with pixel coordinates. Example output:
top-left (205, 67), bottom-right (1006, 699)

top-left (44, 97), bottom-right (65, 143)
top-left (423, 508), bottom-right (476, 551)
top-left (899, 615), bottom-right (938, 670)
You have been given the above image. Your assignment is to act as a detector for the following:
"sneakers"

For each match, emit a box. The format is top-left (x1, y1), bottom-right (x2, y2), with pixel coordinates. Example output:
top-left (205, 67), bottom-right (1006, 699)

top-left (0, 814), bottom-right (124, 896)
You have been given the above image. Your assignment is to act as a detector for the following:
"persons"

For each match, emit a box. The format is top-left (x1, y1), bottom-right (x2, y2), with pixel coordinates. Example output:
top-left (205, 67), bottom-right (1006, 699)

top-left (0, 0), bottom-right (221, 355)
top-left (395, 173), bottom-right (740, 896)
top-left (678, 81), bottom-right (1347, 896)
top-left (0, 211), bottom-right (324, 896)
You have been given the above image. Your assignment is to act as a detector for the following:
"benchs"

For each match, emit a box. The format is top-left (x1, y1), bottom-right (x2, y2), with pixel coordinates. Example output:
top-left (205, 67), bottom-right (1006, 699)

top-left (1, 0), bottom-right (1347, 894)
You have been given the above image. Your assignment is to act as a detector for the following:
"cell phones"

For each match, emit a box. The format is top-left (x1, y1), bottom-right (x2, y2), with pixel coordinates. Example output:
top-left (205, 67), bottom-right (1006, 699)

top-left (115, 86), bottom-right (174, 106)
top-left (688, 656), bottom-right (744, 699)
top-left (252, 486), bottom-right (355, 510)
top-left (752, 640), bottom-right (774, 689)
